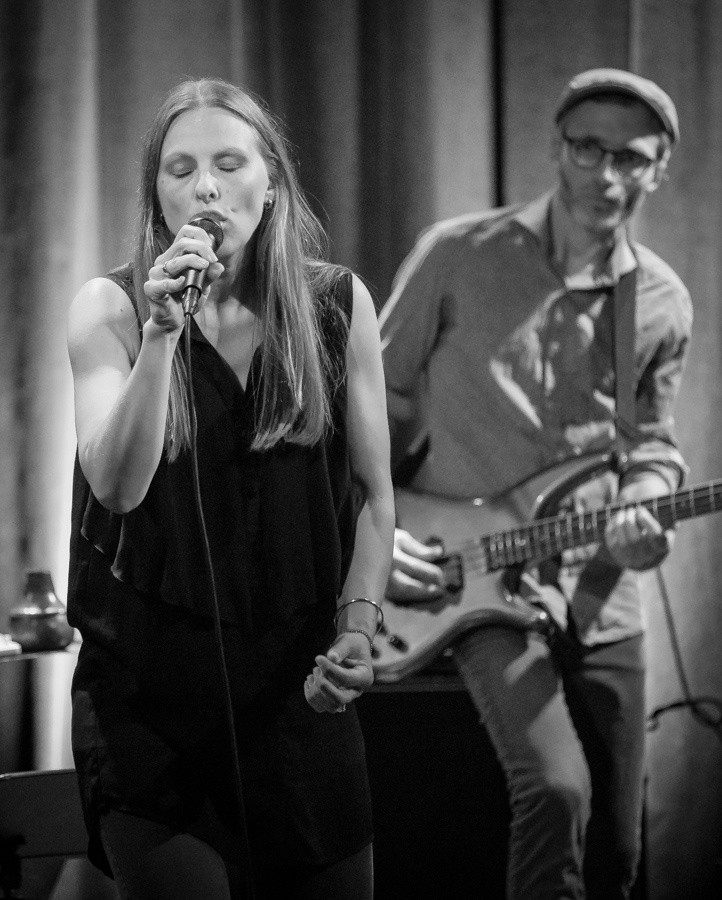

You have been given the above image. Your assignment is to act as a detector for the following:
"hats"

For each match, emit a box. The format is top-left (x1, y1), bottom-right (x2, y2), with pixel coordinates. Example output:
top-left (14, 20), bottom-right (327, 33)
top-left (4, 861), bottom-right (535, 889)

top-left (554, 66), bottom-right (680, 150)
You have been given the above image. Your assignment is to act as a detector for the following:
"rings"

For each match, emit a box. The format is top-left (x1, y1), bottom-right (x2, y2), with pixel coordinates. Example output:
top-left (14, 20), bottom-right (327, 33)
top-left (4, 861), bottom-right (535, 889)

top-left (336, 704), bottom-right (347, 714)
top-left (161, 259), bottom-right (175, 278)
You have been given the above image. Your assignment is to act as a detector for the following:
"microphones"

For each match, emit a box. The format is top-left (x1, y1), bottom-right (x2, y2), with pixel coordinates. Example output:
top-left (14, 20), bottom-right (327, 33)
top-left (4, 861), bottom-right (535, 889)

top-left (175, 215), bottom-right (223, 317)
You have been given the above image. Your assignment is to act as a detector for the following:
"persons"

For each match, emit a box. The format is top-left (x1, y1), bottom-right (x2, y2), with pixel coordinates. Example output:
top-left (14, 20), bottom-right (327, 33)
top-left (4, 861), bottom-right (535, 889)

top-left (59, 79), bottom-right (396, 900)
top-left (370, 62), bottom-right (696, 900)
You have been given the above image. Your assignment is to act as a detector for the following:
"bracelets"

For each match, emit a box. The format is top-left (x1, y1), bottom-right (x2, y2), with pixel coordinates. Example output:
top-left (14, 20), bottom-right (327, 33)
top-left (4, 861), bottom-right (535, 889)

top-left (331, 597), bottom-right (386, 641)
top-left (328, 627), bottom-right (376, 656)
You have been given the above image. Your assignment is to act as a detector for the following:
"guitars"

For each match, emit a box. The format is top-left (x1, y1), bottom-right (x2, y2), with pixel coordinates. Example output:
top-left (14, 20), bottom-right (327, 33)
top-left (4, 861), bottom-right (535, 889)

top-left (344, 449), bottom-right (722, 683)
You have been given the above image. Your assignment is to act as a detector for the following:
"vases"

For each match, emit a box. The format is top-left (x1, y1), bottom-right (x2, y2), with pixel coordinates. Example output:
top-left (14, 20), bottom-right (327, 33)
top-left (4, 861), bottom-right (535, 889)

top-left (10, 571), bottom-right (73, 651)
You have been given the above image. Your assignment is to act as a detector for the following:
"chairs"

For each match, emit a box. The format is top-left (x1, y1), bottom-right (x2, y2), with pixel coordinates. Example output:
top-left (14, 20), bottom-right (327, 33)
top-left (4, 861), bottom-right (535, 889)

top-left (0, 769), bottom-right (92, 898)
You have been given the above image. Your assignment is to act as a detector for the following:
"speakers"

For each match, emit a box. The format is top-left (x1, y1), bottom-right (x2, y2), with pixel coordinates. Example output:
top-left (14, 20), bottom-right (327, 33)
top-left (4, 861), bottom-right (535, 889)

top-left (339, 657), bottom-right (629, 900)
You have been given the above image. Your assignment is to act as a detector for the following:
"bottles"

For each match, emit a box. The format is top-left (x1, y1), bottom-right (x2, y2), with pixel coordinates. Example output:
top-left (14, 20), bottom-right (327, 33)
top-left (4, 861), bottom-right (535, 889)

top-left (10, 569), bottom-right (73, 652)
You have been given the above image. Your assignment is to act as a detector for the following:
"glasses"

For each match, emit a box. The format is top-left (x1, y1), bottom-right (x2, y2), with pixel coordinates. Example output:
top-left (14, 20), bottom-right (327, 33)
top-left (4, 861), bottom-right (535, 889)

top-left (554, 115), bottom-right (661, 180)
